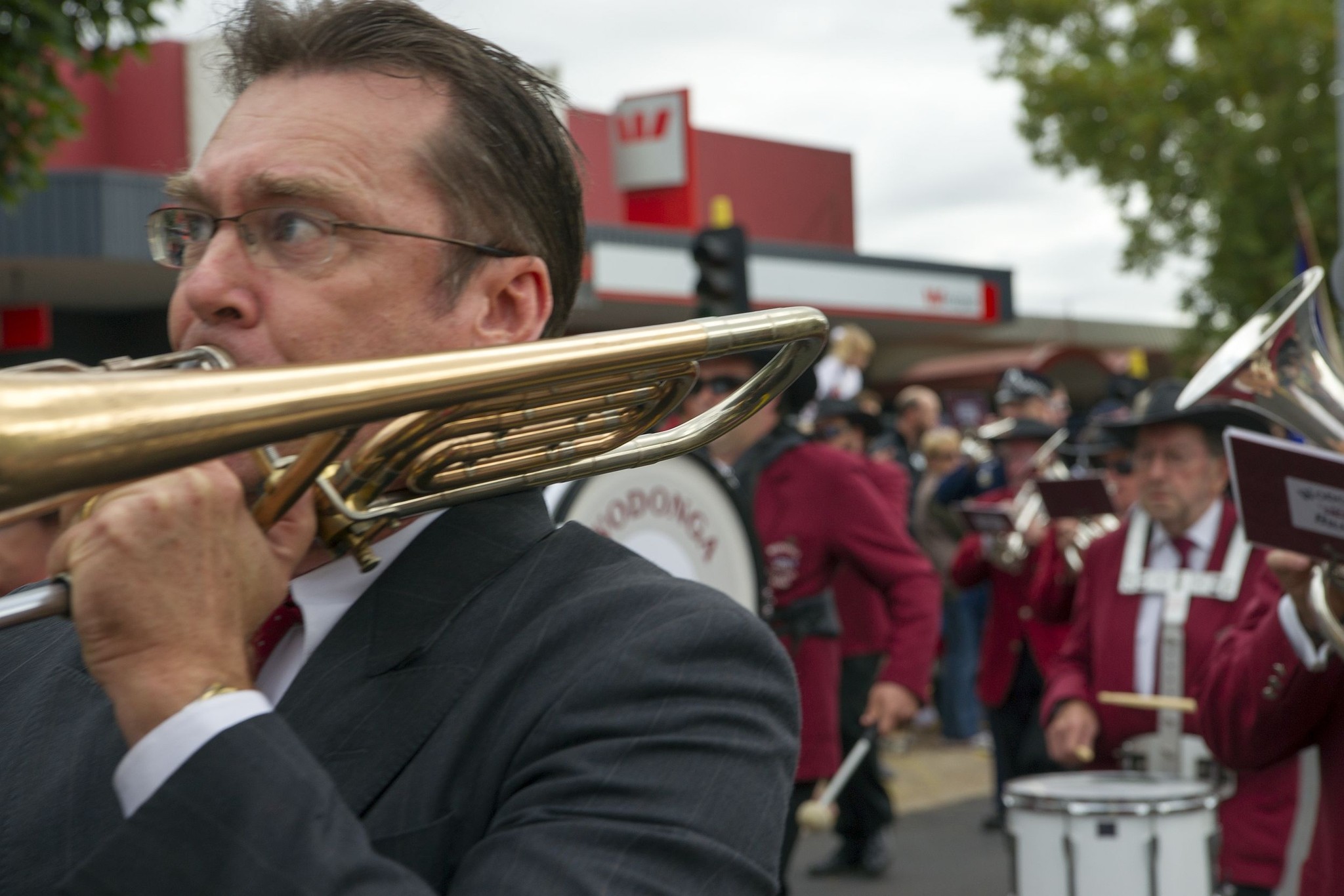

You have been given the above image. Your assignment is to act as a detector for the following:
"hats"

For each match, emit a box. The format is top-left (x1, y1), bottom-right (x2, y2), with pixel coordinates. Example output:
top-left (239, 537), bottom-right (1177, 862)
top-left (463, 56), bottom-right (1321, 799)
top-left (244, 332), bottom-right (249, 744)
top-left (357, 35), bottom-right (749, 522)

top-left (662, 297), bottom-right (1263, 446)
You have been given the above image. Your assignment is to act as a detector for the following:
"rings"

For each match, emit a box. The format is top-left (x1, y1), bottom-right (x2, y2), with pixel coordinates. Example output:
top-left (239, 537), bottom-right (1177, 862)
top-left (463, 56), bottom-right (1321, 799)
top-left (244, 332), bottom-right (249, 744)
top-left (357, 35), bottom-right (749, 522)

top-left (81, 493), bottom-right (101, 521)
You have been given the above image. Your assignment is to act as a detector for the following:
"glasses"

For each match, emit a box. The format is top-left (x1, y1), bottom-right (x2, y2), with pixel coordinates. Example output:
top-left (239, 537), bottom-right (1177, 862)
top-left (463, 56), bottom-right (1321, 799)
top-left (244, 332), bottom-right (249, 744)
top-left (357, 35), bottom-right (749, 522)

top-left (142, 203), bottom-right (529, 271)
top-left (1089, 455), bottom-right (1133, 475)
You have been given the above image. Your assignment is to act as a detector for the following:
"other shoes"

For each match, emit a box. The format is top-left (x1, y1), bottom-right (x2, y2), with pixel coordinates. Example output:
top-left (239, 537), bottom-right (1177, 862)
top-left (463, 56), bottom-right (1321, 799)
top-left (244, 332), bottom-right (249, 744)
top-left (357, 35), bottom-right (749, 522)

top-left (810, 827), bottom-right (897, 879)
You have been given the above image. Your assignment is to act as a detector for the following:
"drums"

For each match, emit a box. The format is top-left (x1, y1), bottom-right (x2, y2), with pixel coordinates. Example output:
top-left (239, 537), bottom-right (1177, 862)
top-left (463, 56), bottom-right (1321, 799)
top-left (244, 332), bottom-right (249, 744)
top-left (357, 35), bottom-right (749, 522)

top-left (999, 768), bottom-right (1229, 896)
top-left (543, 444), bottom-right (775, 624)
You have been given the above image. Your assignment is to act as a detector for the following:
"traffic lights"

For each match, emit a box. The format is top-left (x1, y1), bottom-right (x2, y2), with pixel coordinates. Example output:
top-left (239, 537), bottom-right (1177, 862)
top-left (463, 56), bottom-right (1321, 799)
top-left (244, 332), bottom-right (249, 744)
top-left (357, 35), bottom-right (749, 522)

top-left (691, 226), bottom-right (750, 317)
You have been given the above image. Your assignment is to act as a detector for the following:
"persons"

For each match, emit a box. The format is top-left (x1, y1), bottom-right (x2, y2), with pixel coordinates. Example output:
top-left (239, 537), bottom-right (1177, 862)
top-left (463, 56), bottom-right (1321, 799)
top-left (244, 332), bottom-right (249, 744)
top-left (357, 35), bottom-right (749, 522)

top-left (678, 342), bottom-right (946, 895)
top-left (789, 321), bottom-right (1161, 835)
top-left (1025, 381), bottom-right (1285, 895)
top-left (1194, 537), bottom-right (1343, 896)
top-left (2, 1), bottom-right (799, 895)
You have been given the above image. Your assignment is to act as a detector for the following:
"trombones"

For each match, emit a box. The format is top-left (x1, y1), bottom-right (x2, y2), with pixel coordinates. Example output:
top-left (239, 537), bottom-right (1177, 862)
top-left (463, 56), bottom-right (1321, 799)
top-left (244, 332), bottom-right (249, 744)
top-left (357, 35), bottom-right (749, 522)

top-left (0, 305), bottom-right (833, 625)
